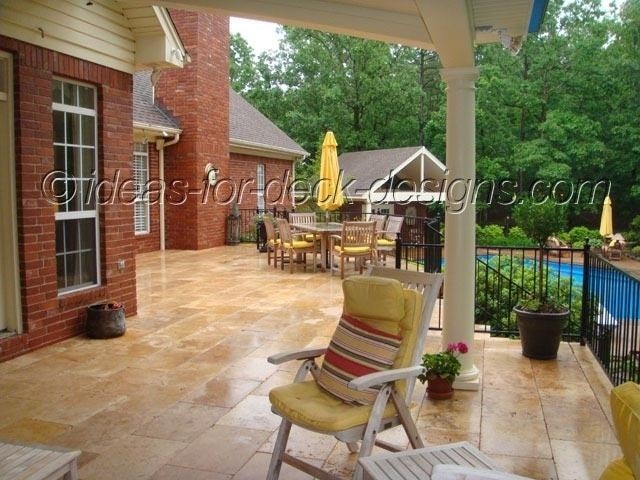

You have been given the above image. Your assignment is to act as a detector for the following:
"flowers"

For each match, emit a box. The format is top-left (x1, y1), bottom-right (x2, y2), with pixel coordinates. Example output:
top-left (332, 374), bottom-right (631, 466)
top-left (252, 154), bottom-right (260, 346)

top-left (418, 341), bottom-right (467, 383)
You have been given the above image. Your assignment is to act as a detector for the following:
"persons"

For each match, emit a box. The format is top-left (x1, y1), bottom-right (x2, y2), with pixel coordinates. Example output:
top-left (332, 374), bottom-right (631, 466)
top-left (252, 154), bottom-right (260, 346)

top-left (316, 127), bottom-right (344, 267)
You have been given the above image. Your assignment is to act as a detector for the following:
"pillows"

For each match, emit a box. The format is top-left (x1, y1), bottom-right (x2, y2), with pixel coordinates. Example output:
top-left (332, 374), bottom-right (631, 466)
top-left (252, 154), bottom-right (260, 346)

top-left (596, 381), bottom-right (640, 480)
top-left (315, 275), bottom-right (404, 406)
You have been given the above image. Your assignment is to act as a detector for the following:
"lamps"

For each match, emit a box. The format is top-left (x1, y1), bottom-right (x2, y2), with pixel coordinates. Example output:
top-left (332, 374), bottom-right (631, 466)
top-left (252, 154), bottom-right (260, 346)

top-left (498, 31), bottom-right (524, 57)
top-left (173, 49), bottom-right (184, 62)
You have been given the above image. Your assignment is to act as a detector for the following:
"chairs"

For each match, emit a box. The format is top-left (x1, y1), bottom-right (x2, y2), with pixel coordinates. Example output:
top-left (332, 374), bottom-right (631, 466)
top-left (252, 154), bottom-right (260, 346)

top-left (546, 238), bottom-right (623, 261)
top-left (266, 265), bottom-right (444, 480)
top-left (261, 211), bottom-right (406, 279)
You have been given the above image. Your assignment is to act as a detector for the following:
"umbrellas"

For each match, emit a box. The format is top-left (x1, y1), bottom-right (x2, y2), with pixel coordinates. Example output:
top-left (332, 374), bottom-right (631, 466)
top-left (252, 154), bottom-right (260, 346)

top-left (599, 195), bottom-right (613, 257)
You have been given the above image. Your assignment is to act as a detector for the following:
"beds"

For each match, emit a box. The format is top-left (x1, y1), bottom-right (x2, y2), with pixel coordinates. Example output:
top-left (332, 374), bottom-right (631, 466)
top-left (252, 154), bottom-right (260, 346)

top-left (356, 441), bottom-right (514, 480)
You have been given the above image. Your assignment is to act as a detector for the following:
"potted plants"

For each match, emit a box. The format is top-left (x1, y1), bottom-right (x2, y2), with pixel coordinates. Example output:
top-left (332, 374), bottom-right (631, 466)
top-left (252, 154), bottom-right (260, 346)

top-left (513, 294), bottom-right (570, 360)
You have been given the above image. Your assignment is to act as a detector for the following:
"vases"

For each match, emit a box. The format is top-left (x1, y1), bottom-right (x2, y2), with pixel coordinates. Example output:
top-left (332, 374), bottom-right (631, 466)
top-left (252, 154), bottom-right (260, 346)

top-left (427, 378), bottom-right (455, 399)
